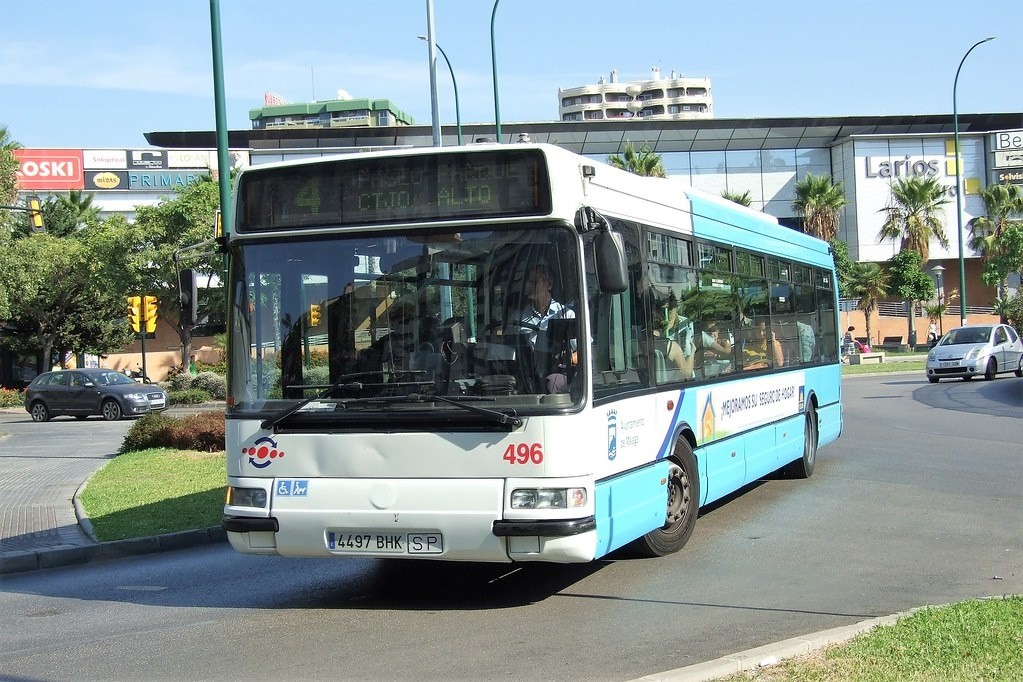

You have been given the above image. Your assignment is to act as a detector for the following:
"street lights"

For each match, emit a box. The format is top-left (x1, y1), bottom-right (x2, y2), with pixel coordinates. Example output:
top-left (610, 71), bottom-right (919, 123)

top-left (418, 36), bottom-right (462, 146)
top-left (931, 265), bottom-right (946, 338)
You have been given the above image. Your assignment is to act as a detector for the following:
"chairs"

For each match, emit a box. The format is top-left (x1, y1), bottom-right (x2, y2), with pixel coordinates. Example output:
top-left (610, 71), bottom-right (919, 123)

top-left (654, 349), bottom-right (667, 383)
top-left (420, 317), bottom-right (471, 338)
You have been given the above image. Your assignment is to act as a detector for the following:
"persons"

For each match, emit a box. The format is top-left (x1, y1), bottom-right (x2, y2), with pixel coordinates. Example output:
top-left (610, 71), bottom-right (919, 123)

top-left (189, 355), bottom-right (197, 376)
top-left (840, 326), bottom-right (857, 364)
top-left (927, 319), bottom-right (937, 351)
top-left (357, 302), bottom-right (444, 396)
top-left (648, 296), bottom-right (817, 383)
top-left (504, 265), bottom-right (593, 396)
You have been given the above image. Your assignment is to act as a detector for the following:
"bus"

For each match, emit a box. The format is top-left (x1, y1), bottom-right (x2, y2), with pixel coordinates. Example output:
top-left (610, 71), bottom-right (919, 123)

top-left (173, 132), bottom-right (842, 563)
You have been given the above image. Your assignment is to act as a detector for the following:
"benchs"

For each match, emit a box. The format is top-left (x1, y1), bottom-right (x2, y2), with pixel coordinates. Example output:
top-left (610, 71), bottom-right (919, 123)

top-left (849, 352), bottom-right (885, 365)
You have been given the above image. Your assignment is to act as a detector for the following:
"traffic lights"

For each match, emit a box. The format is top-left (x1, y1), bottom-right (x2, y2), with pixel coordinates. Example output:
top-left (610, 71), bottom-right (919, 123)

top-left (146, 317), bottom-right (157, 333)
top-left (143, 296), bottom-right (157, 322)
top-left (127, 297), bottom-right (140, 332)
top-left (310, 305), bottom-right (321, 327)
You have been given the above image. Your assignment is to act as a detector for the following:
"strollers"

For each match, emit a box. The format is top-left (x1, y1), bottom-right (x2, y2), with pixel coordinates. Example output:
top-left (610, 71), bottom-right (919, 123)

top-left (854, 341), bottom-right (871, 353)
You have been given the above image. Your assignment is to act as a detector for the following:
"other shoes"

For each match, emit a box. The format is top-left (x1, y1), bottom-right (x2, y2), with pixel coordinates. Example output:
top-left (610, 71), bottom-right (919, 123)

top-left (928, 347), bottom-right (931, 351)
top-left (841, 358), bottom-right (845, 363)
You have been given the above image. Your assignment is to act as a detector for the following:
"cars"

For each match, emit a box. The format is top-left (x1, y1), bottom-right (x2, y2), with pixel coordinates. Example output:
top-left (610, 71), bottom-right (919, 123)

top-left (926, 324), bottom-right (1023, 383)
top-left (24, 368), bottom-right (169, 421)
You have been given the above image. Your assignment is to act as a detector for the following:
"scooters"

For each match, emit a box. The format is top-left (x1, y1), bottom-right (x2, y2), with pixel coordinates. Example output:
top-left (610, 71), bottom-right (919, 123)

top-left (122, 363), bottom-right (151, 385)
